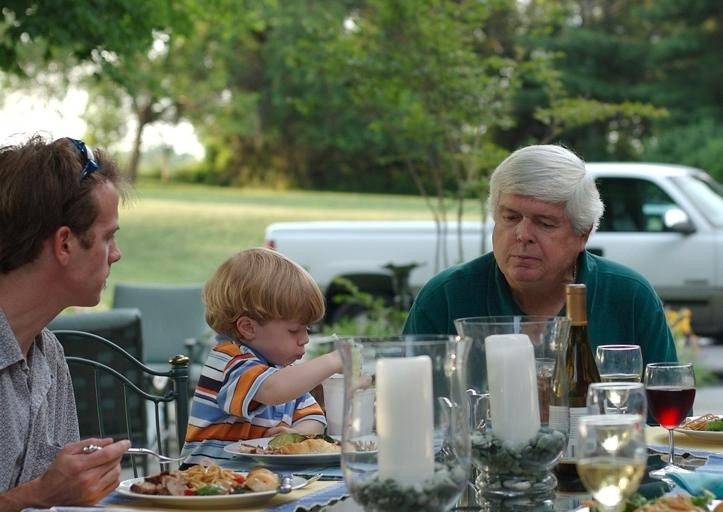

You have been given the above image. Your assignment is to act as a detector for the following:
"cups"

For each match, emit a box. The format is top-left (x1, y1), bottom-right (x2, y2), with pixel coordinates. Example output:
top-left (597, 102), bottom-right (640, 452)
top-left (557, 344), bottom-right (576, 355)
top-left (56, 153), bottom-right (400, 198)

top-left (534, 356), bottom-right (556, 427)
top-left (575, 344), bottom-right (649, 511)
top-left (321, 371), bottom-right (376, 438)
top-left (453, 316), bottom-right (570, 496)
top-left (334, 333), bottom-right (474, 512)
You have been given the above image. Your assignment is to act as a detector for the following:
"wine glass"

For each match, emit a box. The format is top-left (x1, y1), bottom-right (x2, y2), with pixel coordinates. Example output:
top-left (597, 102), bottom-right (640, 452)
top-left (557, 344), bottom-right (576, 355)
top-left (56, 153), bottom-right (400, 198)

top-left (643, 361), bottom-right (697, 465)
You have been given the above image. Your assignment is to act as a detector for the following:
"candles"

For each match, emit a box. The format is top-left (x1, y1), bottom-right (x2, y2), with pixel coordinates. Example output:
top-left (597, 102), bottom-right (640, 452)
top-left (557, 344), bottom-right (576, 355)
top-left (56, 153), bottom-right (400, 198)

top-left (484, 333), bottom-right (540, 448)
top-left (372, 354), bottom-right (433, 490)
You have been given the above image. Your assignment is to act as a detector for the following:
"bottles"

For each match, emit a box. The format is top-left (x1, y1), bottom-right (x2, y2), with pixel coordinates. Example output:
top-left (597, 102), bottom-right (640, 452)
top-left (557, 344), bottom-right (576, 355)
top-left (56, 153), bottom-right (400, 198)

top-left (548, 282), bottom-right (603, 493)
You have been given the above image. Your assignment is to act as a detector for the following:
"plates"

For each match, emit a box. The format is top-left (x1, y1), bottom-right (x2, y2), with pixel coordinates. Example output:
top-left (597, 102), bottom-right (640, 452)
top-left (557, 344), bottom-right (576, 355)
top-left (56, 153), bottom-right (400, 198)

top-left (659, 414), bottom-right (723, 443)
top-left (114, 471), bottom-right (307, 510)
top-left (223, 435), bottom-right (379, 466)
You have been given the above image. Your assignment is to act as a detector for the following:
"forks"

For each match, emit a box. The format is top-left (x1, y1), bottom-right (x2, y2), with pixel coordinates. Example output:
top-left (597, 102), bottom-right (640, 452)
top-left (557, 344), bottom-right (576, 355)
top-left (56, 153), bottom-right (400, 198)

top-left (82, 444), bottom-right (185, 463)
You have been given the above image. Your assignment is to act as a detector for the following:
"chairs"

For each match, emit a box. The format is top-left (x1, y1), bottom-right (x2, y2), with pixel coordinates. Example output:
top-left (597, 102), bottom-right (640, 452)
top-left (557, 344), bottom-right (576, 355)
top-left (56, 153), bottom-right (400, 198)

top-left (46, 282), bottom-right (215, 481)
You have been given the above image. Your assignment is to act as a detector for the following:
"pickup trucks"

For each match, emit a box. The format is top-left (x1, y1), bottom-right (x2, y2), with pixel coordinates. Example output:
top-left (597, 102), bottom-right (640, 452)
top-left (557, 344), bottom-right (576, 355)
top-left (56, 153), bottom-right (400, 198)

top-left (257, 154), bottom-right (723, 347)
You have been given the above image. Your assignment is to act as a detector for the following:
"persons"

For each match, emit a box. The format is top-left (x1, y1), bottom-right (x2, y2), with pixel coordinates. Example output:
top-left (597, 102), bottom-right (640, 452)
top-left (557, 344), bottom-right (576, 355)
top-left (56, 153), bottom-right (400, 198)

top-left (178, 247), bottom-right (366, 469)
top-left (0, 130), bottom-right (133, 512)
top-left (402, 141), bottom-right (692, 429)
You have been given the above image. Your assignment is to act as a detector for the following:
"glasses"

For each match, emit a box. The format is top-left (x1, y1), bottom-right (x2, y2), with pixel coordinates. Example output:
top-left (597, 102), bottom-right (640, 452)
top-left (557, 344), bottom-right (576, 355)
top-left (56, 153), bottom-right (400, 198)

top-left (66, 136), bottom-right (98, 184)
